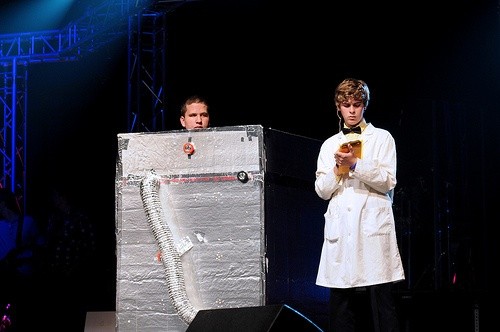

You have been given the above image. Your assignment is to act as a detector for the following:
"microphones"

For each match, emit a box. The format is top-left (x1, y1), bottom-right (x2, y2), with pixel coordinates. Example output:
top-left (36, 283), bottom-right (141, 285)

top-left (337, 109), bottom-right (341, 120)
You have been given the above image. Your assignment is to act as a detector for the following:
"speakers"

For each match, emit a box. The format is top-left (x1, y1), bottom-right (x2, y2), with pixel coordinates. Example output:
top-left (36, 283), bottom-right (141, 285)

top-left (185, 305), bottom-right (325, 332)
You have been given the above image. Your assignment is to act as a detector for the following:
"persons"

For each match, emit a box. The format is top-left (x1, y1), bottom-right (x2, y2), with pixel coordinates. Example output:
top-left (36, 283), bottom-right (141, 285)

top-left (315, 78), bottom-right (406, 332)
top-left (180, 96), bottom-right (211, 130)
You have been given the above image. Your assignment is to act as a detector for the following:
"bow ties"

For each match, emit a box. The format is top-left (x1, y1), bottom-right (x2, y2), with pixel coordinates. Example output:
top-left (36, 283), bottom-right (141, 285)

top-left (342, 126), bottom-right (361, 135)
